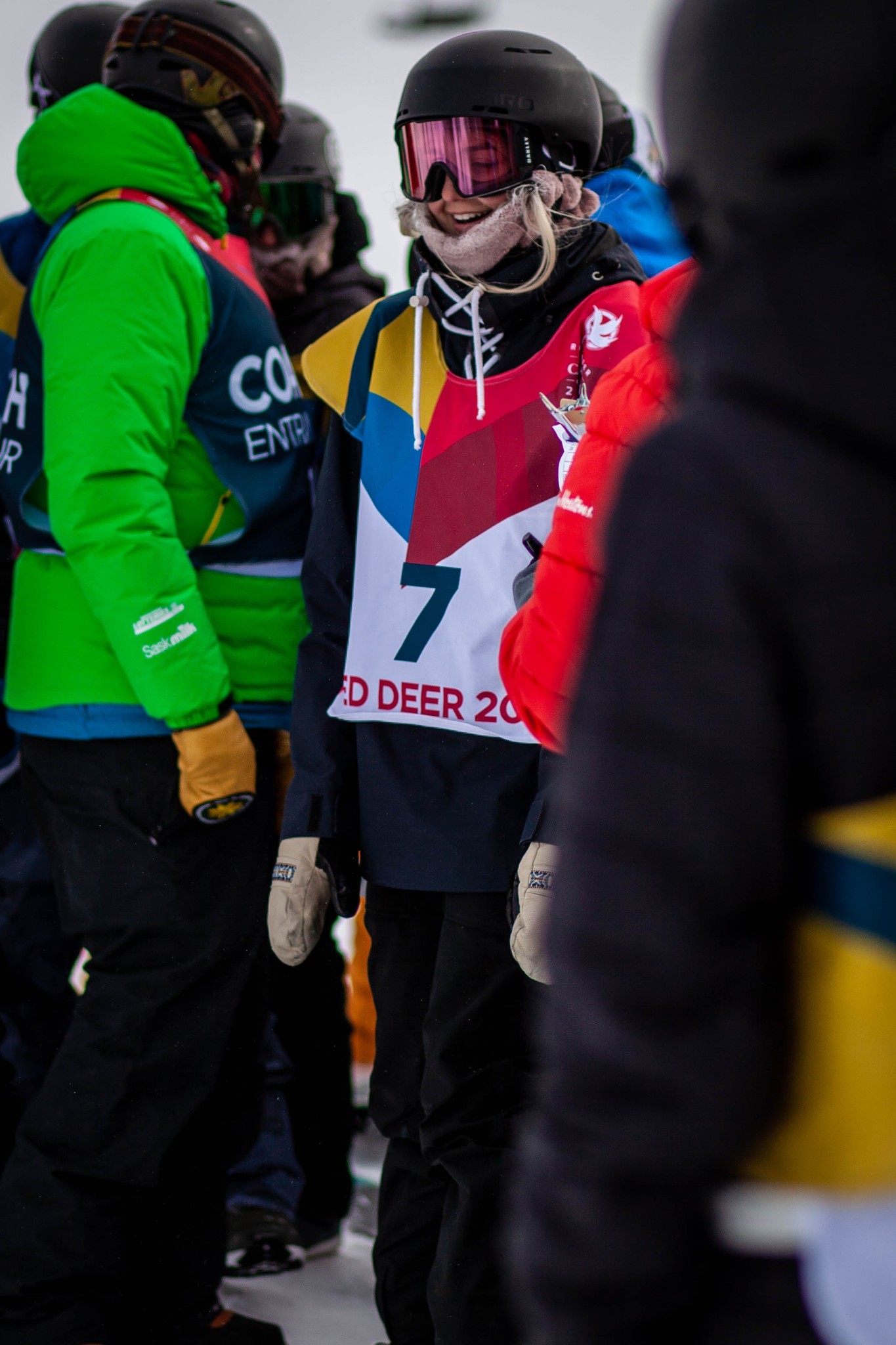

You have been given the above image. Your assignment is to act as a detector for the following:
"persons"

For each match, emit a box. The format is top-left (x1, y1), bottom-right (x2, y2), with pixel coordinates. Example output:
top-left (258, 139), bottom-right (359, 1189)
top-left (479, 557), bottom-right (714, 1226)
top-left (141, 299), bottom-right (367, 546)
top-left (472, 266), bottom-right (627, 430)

top-left (268, 32), bottom-right (691, 1345)
top-left (0, 1), bottom-right (388, 1345)
top-left (497, 0), bottom-right (895, 1345)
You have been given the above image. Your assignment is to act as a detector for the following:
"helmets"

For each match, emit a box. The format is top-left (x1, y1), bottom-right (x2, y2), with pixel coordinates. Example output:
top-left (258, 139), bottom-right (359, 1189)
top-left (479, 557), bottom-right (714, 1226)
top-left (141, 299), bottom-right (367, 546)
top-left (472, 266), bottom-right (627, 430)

top-left (27, 4), bottom-right (130, 119)
top-left (393, 30), bottom-right (603, 171)
top-left (264, 104), bottom-right (335, 179)
top-left (659, 0), bottom-right (896, 217)
top-left (101, 1), bottom-right (284, 168)
top-left (590, 72), bottom-right (633, 156)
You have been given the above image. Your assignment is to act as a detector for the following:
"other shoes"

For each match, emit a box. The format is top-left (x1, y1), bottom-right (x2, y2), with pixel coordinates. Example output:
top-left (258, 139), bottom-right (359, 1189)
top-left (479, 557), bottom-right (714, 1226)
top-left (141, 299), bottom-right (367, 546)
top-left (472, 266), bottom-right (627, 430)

top-left (298, 1216), bottom-right (341, 1259)
top-left (193, 1304), bottom-right (285, 1345)
top-left (224, 1204), bottom-right (306, 1278)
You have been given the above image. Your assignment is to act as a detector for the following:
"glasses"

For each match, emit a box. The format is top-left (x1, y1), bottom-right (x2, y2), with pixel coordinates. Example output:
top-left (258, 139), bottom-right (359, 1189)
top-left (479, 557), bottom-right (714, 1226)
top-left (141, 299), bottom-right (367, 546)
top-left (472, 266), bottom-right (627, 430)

top-left (399, 114), bottom-right (542, 203)
top-left (238, 175), bottom-right (328, 247)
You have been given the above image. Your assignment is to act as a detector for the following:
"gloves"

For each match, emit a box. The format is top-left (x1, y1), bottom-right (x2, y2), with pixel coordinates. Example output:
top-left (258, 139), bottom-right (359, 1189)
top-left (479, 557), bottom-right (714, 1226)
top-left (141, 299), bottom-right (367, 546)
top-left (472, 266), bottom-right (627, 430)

top-left (170, 708), bottom-right (257, 825)
top-left (509, 840), bottom-right (567, 987)
top-left (266, 838), bottom-right (335, 967)
top-left (248, 727), bottom-right (292, 829)
